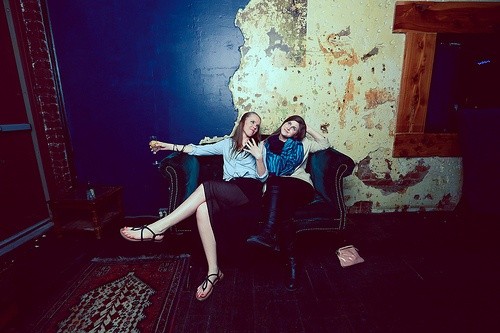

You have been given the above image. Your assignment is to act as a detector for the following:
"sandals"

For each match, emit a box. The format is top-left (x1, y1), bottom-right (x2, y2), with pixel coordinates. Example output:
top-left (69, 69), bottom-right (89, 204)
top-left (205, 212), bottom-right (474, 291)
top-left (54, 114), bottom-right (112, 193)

top-left (195, 268), bottom-right (224, 301)
top-left (119, 224), bottom-right (164, 242)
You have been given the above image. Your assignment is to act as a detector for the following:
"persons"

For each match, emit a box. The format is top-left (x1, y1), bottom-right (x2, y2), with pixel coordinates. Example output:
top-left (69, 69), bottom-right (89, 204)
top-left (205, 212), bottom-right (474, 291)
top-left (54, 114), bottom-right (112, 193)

top-left (119, 112), bottom-right (269, 301)
top-left (247, 114), bottom-right (332, 291)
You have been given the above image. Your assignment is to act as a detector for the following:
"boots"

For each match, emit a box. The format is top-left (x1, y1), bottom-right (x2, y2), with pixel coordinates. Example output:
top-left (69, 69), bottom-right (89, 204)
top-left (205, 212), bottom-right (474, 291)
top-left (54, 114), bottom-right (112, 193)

top-left (285, 256), bottom-right (302, 291)
top-left (245, 186), bottom-right (280, 247)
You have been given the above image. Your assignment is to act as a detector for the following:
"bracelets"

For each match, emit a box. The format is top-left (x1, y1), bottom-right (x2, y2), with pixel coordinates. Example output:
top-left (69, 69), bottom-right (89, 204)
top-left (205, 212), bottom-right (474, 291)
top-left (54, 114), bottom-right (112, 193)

top-left (172, 144), bottom-right (186, 152)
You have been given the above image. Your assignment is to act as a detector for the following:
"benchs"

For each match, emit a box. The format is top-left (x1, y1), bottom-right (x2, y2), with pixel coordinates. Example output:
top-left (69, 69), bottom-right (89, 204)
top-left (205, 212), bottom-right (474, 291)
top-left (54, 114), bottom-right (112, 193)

top-left (157, 135), bottom-right (357, 283)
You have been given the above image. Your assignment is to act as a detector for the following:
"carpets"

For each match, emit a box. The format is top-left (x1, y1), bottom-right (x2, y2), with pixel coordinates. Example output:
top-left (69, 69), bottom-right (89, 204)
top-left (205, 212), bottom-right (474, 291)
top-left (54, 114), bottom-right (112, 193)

top-left (29, 251), bottom-right (191, 333)
